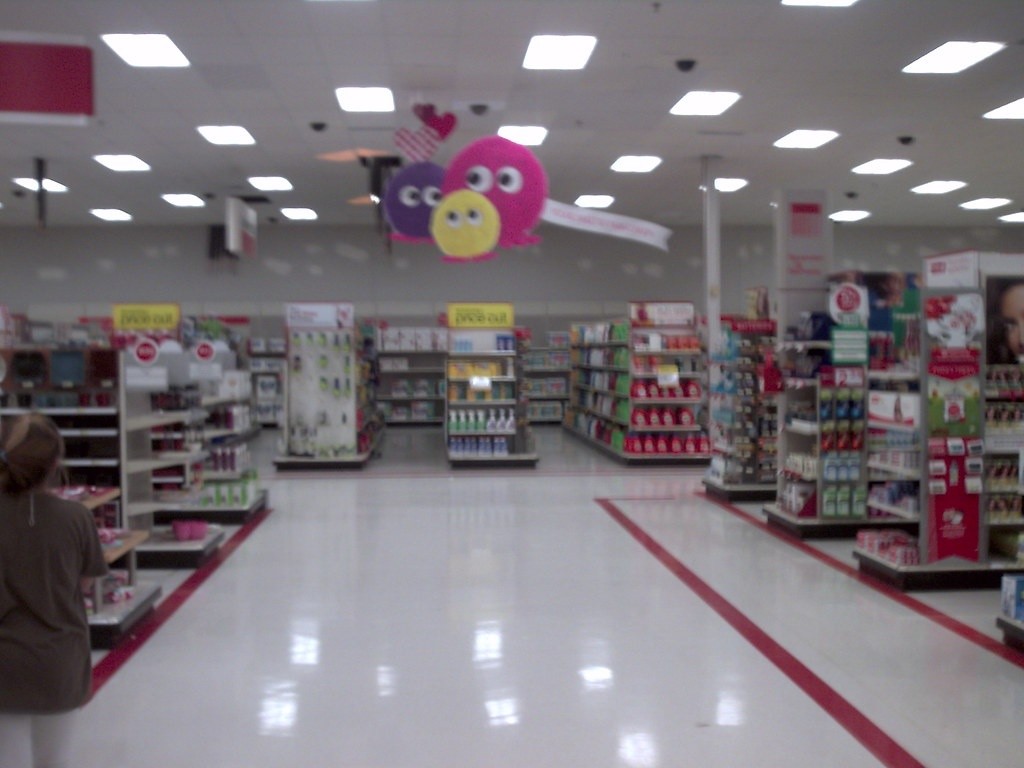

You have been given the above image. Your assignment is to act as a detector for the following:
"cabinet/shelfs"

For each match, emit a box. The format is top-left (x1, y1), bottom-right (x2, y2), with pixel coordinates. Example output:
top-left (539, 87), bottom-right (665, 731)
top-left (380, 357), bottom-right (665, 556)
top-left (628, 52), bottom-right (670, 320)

top-left (702, 247), bottom-right (1024, 652)
top-left (376, 291), bottom-right (717, 473)
top-left (3, 297), bottom-right (380, 705)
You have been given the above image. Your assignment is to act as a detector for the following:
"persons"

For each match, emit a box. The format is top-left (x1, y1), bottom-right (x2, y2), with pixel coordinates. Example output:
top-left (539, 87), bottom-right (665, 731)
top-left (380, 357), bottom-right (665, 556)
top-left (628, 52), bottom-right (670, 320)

top-left (876, 272), bottom-right (906, 307)
top-left (0, 412), bottom-right (108, 768)
top-left (986, 277), bottom-right (1024, 365)
